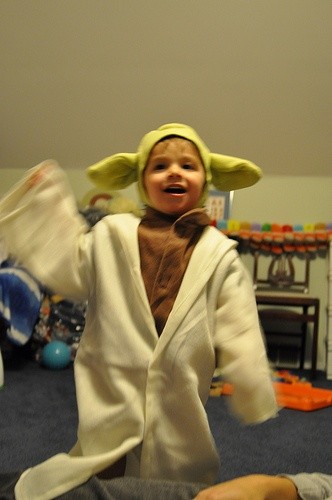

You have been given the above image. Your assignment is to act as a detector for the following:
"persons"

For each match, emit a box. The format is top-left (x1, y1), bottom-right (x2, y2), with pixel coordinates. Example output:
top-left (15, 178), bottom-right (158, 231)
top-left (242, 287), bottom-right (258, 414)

top-left (0, 123), bottom-right (277, 500)
top-left (0, 471), bottom-right (332, 500)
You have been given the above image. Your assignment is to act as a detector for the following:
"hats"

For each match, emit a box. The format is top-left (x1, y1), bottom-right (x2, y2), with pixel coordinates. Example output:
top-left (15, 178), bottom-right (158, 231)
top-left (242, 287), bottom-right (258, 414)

top-left (86, 122), bottom-right (261, 213)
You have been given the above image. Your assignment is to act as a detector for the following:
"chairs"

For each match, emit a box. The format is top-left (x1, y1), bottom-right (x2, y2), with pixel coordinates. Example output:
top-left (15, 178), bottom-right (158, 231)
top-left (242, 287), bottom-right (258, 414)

top-left (249, 245), bottom-right (321, 383)
